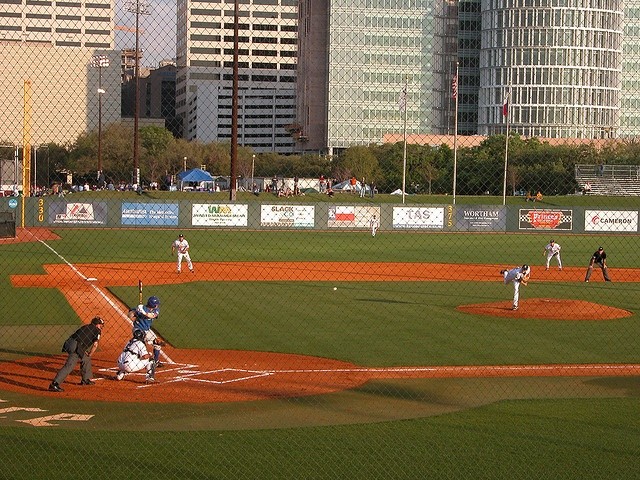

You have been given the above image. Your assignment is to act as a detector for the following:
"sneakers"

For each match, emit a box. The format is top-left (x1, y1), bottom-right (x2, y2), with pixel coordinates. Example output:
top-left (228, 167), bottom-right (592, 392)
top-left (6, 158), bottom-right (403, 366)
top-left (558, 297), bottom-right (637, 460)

top-left (81, 379), bottom-right (95, 385)
top-left (586, 279), bottom-right (588, 283)
top-left (49, 384), bottom-right (64, 392)
top-left (500, 270), bottom-right (507, 274)
top-left (513, 306), bottom-right (518, 311)
top-left (146, 373), bottom-right (155, 381)
top-left (178, 271), bottom-right (181, 274)
top-left (545, 268), bottom-right (549, 270)
top-left (156, 360), bottom-right (163, 367)
top-left (191, 270), bottom-right (194, 273)
top-left (117, 372), bottom-right (125, 380)
top-left (559, 268), bottom-right (562, 271)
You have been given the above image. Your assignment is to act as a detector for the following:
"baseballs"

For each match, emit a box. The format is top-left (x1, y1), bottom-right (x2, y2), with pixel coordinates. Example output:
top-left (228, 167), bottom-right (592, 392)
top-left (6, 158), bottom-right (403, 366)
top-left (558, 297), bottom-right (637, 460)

top-left (333, 287), bottom-right (337, 290)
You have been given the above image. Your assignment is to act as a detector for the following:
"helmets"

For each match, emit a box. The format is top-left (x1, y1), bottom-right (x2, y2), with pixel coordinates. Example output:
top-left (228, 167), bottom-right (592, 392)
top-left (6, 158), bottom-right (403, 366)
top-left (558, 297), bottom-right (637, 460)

top-left (179, 234), bottom-right (183, 237)
top-left (599, 247), bottom-right (603, 251)
top-left (133, 329), bottom-right (146, 341)
top-left (523, 265), bottom-right (528, 270)
top-left (92, 318), bottom-right (104, 324)
top-left (550, 240), bottom-right (554, 243)
top-left (148, 297), bottom-right (159, 308)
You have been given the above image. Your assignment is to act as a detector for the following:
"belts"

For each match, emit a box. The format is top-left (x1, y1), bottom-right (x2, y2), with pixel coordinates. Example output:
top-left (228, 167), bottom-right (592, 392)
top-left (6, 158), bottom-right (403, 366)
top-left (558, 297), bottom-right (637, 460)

top-left (118, 361), bottom-right (129, 365)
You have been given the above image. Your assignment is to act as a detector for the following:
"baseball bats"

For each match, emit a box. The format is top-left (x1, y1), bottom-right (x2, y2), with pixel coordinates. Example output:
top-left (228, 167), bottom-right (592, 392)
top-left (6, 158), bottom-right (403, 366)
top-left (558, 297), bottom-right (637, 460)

top-left (139, 281), bottom-right (143, 304)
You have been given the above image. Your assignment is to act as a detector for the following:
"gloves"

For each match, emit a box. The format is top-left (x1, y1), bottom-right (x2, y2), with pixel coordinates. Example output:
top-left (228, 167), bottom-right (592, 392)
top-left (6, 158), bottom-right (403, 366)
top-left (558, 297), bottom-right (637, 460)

top-left (140, 309), bottom-right (146, 316)
top-left (136, 305), bottom-right (144, 312)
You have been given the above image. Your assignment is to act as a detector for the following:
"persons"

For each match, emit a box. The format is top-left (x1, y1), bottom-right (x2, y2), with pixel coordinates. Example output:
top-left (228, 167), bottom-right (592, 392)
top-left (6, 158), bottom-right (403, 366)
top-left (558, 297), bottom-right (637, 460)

top-left (128, 296), bottom-right (163, 367)
top-left (113, 330), bottom-right (155, 381)
top-left (350, 177), bottom-right (356, 195)
top-left (28, 179), bottom-right (160, 197)
top-left (190, 182), bottom-right (253, 193)
top-left (370, 181), bottom-right (375, 197)
top-left (253, 174), bottom-right (300, 197)
top-left (536, 191), bottom-right (542, 201)
top-left (172, 234), bottom-right (194, 273)
top-left (543, 239), bottom-right (562, 271)
top-left (585, 247), bottom-right (610, 282)
top-left (319, 175), bottom-right (334, 198)
top-left (584, 182), bottom-right (591, 191)
top-left (48, 317), bottom-right (105, 391)
top-left (370, 215), bottom-right (378, 237)
top-left (360, 177), bottom-right (366, 197)
top-left (525, 191), bottom-right (535, 202)
top-left (499, 264), bottom-right (531, 310)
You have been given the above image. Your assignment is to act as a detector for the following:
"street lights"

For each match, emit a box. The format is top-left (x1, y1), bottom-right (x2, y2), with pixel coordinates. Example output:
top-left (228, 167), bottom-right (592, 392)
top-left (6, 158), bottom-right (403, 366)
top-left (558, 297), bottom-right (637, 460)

top-left (91, 55), bottom-right (110, 181)
top-left (124, 1), bottom-right (151, 184)
